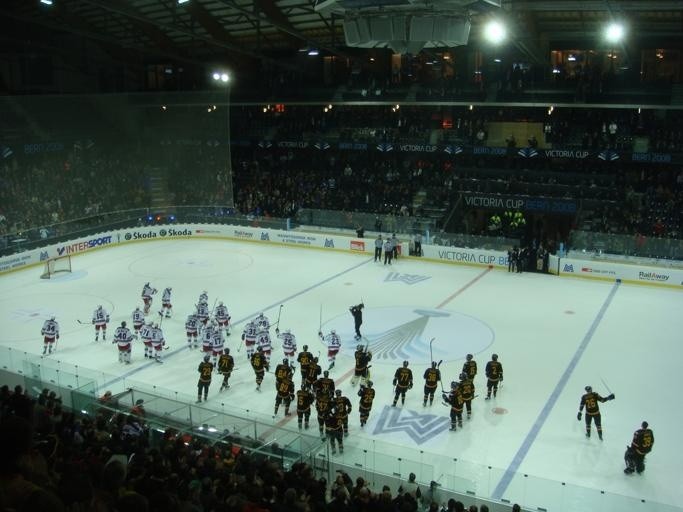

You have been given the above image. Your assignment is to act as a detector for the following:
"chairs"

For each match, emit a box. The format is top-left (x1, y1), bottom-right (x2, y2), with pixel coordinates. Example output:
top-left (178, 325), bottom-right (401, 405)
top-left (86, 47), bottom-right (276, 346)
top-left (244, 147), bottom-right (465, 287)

top-left (0, 103), bottom-right (683, 258)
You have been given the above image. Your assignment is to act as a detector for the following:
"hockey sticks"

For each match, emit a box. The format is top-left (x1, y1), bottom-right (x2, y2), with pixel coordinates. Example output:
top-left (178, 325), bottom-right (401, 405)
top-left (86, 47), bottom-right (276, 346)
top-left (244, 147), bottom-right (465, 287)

top-left (77, 320), bottom-right (91, 324)
top-left (364, 337), bottom-right (369, 353)
top-left (430, 338), bottom-right (434, 362)
top-left (437, 360), bottom-right (444, 390)
top-left (158, 312), bottom-right (163, 328)
top-left (238, 342), bottom-right (242, 352)
top-left (365, 365), bottom-right (371, 379)
top-left (277, 305), bottom-right (283, 326)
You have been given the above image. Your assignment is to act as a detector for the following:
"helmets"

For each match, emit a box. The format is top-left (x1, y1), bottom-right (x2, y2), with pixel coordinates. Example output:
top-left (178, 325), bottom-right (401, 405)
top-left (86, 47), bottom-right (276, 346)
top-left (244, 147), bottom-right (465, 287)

top-left (204, 345), bottom-right (498, 406)
top-left (585, 386), bottom-right (593, 392)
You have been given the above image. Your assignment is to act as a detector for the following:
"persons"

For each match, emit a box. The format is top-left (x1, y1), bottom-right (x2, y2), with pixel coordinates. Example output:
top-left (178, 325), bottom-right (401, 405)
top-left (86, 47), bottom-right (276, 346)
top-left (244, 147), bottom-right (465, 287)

top-left (392, 62), bottom-right (399, 82)
top-left (497, 61), bottom-right (532, 92)
